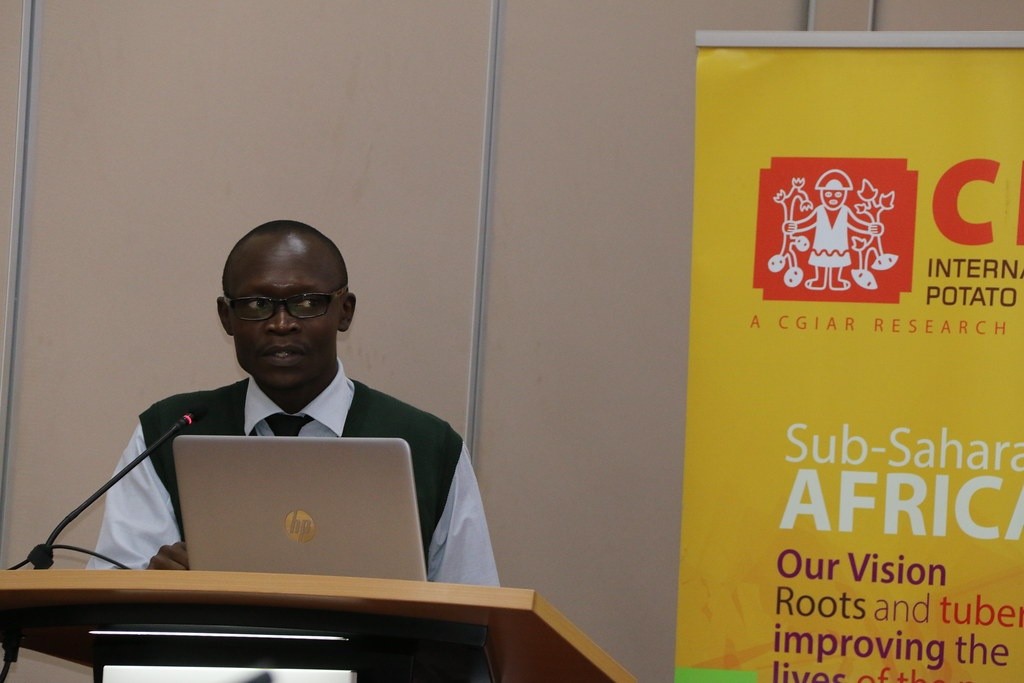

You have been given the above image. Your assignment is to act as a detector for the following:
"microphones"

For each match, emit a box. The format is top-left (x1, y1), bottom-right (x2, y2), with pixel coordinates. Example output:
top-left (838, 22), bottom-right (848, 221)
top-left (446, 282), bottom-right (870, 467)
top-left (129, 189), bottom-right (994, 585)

top-left (32, 403), bottom-right (209, 571)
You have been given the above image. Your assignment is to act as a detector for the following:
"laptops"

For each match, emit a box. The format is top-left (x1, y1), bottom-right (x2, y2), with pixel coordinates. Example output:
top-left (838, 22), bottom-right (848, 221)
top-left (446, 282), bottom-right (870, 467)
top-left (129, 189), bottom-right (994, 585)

top-left (171, 435), bottom-right (428, 583)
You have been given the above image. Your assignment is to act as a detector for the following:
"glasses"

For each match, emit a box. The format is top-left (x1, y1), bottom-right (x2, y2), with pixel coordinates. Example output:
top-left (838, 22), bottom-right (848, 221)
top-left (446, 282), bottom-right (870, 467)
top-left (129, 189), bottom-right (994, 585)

top-left (221, 280), bottom-right (350, 323)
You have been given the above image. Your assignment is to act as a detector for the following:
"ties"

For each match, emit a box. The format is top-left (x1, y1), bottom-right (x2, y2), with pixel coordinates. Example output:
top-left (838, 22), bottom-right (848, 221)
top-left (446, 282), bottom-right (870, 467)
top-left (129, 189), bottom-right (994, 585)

top-left (264, 414), bottom-right (315, 437)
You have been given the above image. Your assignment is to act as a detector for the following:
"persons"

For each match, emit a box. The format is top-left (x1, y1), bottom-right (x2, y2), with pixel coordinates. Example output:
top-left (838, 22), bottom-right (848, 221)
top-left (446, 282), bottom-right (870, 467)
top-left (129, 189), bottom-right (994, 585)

top-left (88, 219), bottom-right (507, 589)
